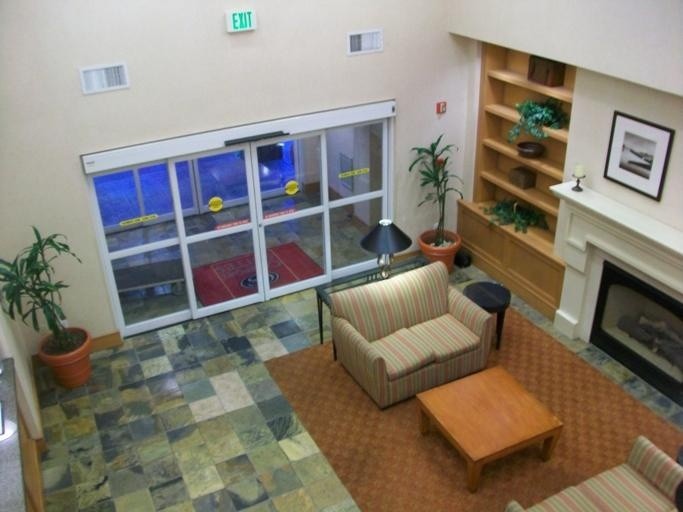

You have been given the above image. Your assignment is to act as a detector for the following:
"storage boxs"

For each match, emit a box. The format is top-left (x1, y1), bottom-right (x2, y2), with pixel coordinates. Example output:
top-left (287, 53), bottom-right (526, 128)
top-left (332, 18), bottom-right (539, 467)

top-left (527, 55), bottom-right (565, 86)
top-left (510, 168), bottom-right (536, 191)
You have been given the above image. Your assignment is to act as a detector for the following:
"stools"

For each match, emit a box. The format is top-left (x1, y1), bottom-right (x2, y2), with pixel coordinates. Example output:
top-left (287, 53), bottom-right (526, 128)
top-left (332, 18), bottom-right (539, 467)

top-left (462, 282), bottom-right (511, 349)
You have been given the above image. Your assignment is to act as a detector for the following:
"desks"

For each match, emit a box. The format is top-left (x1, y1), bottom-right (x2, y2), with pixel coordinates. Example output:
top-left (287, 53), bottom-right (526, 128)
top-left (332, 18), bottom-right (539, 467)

top-left (315, 257), bottom-right (437, 361)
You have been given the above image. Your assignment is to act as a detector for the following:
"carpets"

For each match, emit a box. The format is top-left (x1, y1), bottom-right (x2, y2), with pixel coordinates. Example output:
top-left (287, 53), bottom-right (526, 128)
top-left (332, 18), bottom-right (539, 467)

top-left (263, 307), bottom-right (683, 512)
top-left (192, 242), bottom-right (324, 305)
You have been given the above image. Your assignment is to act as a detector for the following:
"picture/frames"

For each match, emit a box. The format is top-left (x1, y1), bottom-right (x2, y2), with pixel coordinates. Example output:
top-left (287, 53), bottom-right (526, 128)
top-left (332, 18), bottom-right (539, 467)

top-left (604, 110), bottom-right (676, 201)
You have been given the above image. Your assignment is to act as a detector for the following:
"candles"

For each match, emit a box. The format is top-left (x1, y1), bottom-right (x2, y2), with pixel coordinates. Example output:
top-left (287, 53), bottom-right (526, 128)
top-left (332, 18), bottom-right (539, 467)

top-left (574, 166), bottom-right (584, 177)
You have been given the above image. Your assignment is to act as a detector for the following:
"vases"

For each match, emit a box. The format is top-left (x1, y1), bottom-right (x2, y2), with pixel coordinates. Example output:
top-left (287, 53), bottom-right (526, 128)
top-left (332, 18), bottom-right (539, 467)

top-left (418, 229), bottom-right (461, 274)
top-left (515, 142), bottom-right (545, 156)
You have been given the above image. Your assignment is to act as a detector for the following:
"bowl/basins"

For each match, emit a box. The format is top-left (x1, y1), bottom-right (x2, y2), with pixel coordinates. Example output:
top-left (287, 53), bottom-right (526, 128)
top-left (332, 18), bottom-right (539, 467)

top-left (516, 141), bottom-right (545, 158)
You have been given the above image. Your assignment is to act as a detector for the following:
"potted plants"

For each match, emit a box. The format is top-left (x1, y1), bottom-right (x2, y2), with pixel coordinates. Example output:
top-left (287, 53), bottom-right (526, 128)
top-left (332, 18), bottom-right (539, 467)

top-left (0, 226), bottom-right (92, 387)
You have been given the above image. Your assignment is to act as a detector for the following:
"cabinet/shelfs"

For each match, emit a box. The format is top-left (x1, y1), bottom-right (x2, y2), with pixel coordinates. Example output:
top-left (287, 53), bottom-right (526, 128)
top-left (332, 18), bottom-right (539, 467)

top-left (457, 41), bottom-right (577, 320)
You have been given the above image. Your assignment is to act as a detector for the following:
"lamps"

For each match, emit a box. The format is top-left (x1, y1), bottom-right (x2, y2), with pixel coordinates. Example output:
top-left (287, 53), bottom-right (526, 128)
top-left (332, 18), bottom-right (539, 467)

top-left (358, 218), bottom-right (413, 281)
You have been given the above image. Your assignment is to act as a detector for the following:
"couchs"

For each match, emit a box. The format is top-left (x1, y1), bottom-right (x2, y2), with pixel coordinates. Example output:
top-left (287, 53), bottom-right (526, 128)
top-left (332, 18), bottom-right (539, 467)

top-left (328, 261), bottom-right (493, 410)
top-left (506, 435), bottom-right (683, 512)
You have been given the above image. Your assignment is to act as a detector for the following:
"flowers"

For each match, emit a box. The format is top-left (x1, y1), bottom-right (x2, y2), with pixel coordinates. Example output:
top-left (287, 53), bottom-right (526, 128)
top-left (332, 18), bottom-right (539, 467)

top-left (409, 133), bottom-right (464, 245)
